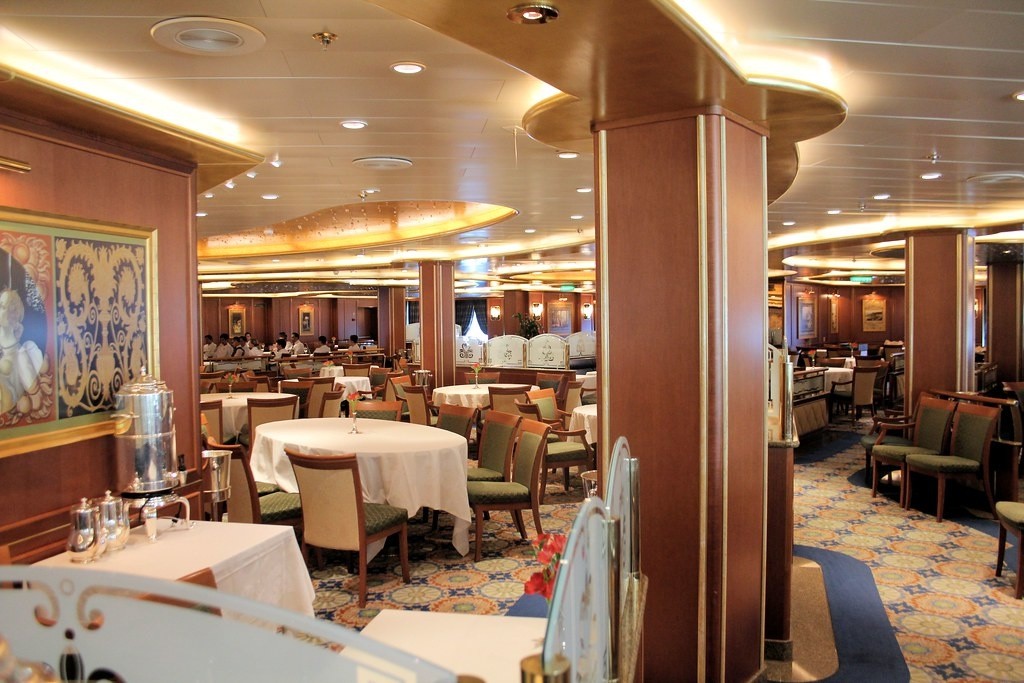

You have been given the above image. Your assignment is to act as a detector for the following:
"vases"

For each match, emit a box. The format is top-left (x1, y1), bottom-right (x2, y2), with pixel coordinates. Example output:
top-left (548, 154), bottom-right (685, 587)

top-left (351, 410), bottom-right (359, 435)
top-left (474, 375), bottom-right (479, 388)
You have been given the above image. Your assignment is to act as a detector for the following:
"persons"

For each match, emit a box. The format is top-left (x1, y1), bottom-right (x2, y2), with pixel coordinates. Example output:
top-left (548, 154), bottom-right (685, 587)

top-left (203, 331), bottom-right (304, 359)
top-left (348, 335), bottom-right (360, 351)
top-left (313, 336), bottom-right (332, 354)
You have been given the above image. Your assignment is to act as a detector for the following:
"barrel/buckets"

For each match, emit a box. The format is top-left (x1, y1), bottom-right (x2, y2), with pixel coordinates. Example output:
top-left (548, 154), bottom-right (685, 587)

top-left (112, 365), bottom-right (186, 498)
top-left (200, 449), bottom-right (233, 504)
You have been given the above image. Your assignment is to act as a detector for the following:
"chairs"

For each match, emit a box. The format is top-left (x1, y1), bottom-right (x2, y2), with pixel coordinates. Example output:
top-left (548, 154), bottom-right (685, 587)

top-left (795, 345), bottom-right (1024, 600)
top-left (198, 365), bottom-right (595, 600)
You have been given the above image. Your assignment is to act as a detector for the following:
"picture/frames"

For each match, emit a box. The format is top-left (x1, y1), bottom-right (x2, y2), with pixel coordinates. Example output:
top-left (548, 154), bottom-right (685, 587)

top-left (0, 205), bottom-right (161, 459)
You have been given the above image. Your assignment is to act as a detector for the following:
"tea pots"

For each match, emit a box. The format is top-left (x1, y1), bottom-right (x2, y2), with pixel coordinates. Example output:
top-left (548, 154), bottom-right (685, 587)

top-left (96, 490), bottom-right (132, 554)
top-left (65, 496), bottom-right (109, 561)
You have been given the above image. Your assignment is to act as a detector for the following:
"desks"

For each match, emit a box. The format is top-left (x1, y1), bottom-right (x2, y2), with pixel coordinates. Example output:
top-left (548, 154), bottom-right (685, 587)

top-left (805, 366), bottom-right (854, 414)
top-left (201, 392), bottom-right (300, 434)
top-left (250, 417), bottom-right (472, 557)
top-left (432, 384), bottom-right (540, 409)
top-left (278, 376), bottom-right (373, 417)
top-left (338, 608), bottom-right (548, 683)
top-left (20, 518), bottom-right (315, 638)
top-left (319, 365), bottom-right (379, 377)
top-left (567, 403), bottom-right (597, 445)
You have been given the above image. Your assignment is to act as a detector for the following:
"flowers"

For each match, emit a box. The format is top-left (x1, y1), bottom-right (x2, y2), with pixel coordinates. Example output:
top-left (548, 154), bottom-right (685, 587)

top-left (847, 342), bottom-right (858, 349)
top-left (525, 532), bottom-right (568, 599)
top-left (220, 373), bottom-right (240, 385)
top-left (322, 360), bottom-right (334, 367)
top-left (807, 349), bottom-right (816, 357)
top-left (346, 389), bottom-right (363, 412)
top-left (345, 350), bottom-right (353, 358)
top-left (471, 363), bottom-right (482, 375)
